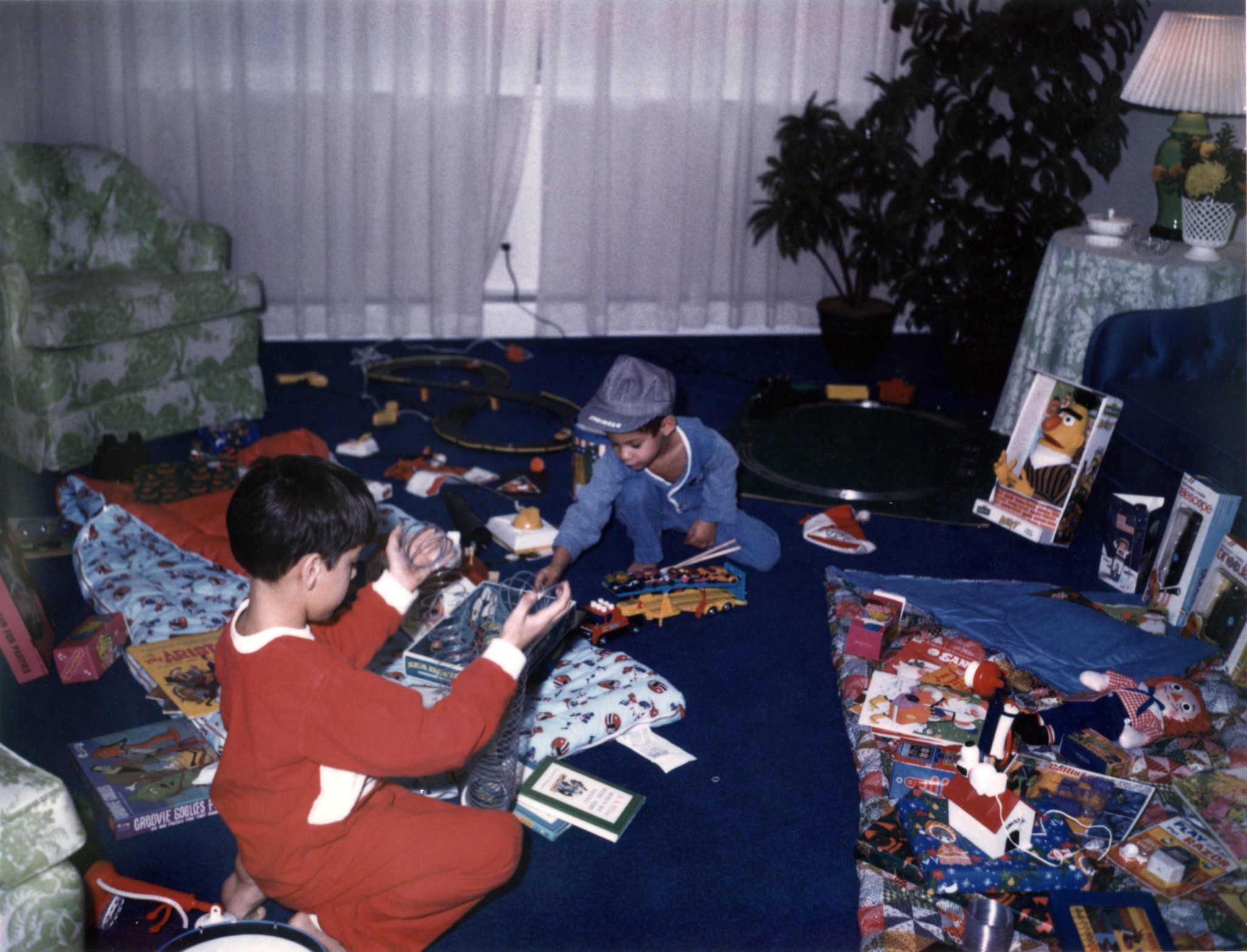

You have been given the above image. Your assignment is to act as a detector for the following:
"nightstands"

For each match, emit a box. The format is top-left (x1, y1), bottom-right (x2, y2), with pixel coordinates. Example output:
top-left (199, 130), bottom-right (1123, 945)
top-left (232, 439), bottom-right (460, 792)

top-left (988, 227), bottom-right (1247, 437)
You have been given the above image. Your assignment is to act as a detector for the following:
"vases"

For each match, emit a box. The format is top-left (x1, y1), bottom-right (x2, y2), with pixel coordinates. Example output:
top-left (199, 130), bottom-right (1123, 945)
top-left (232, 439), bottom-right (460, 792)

top-left (1180, 196), bottom-right (1237, 261)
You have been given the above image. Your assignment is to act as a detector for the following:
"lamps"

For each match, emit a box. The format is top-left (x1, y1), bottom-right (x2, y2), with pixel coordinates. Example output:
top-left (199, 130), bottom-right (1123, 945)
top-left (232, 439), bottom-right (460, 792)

top-left (1120, 8), bottom-right (1246, 241)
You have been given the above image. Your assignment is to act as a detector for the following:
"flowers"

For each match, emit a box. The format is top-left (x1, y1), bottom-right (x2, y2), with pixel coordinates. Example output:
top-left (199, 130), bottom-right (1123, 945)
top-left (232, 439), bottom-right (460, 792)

top-left (1150, 120), bottom-right (1247, 214)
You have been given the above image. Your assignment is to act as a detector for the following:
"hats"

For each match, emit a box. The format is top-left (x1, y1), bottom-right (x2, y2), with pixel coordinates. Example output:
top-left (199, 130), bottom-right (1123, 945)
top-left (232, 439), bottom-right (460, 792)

top-left (579, 355), bottom-right (675, 431)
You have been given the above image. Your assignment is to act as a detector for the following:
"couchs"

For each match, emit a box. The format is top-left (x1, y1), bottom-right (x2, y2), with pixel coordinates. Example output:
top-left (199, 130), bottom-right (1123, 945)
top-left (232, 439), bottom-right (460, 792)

top-left (1082, 294), bottom-right (1247, 536)
top-left (0, 144), bottom-right (267, 474)
top-left (0, 743), bottom-right (87, 952)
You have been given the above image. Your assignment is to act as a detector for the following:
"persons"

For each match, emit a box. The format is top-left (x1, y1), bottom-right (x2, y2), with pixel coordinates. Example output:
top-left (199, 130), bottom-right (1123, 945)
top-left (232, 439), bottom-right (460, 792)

top-left (207, 452), bottom-right (570, 952)
top-left (535, 353), bottom-right (780, 594)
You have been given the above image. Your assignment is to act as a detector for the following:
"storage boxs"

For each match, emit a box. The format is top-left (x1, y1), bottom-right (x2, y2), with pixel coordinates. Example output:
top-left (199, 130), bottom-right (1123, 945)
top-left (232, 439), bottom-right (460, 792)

top-left (846, 593), bottom-right (903, 660)
top-left (67, 718), bottom-right (219, 840)
top-left (972, 369), bottom-right (1123, 550)
top-left (1141, 470), bottom-right (1242, 627)
top-left (52, 611), bottom-right (129, 684)
top-left (0, 522), bottom-right (55, 684)
top-left (402, 580), bottom-right (576, 689)
top-left (889, 737), bottom-right (997, 798)
top-left (1179, 534), bottom-right (1247, 688)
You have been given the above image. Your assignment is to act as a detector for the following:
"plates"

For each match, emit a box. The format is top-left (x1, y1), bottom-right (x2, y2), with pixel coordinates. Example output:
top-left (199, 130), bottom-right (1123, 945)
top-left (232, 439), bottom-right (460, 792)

top-left (1085, 236), bottom-right (1124, 247)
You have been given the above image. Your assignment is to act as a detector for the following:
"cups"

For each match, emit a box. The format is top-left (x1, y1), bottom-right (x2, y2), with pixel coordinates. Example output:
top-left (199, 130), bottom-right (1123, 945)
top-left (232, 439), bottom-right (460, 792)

top-left (963, 898), bottom-right (1015, 952)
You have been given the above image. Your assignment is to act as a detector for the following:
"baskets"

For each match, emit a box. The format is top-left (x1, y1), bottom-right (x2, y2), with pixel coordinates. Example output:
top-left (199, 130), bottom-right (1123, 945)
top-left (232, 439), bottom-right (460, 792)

top-left (1179, 194), bottom-right (1237, 262)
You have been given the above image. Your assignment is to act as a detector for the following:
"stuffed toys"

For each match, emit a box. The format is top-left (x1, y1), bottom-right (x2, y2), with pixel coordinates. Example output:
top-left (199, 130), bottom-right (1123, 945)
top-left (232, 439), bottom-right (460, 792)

top-left (992, 404), bottom-right (1088, 508)
top-left (1010, 670), bottom-right (1207, 747)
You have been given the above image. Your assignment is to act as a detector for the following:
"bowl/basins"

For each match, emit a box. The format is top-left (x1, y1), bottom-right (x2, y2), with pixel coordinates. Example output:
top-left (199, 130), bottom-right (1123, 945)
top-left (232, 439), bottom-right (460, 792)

top-left (1086, 212), bottom-right (1135, 235)
top-left (1132, 237), bottom-right (1172, 256)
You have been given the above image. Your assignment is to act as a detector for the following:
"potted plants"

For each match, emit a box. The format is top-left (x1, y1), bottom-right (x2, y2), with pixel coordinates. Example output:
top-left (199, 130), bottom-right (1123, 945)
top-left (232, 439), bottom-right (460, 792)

top-left (746, 90), bottom-right (900, 367)
